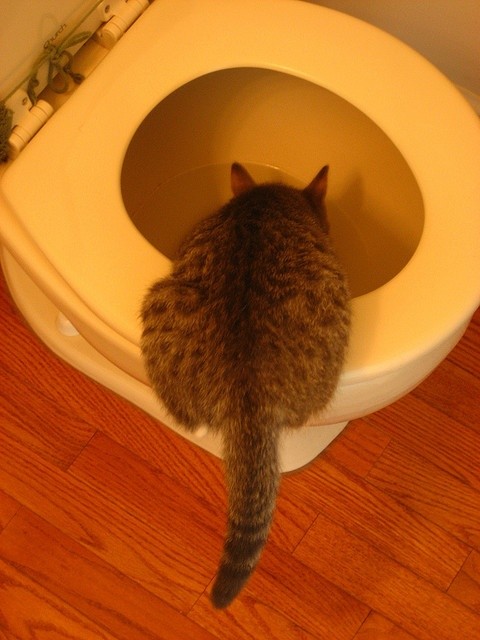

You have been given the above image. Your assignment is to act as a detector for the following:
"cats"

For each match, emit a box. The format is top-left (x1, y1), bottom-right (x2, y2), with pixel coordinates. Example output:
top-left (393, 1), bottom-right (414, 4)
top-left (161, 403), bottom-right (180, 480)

top-left (137, 161), bottom-right (353, 609)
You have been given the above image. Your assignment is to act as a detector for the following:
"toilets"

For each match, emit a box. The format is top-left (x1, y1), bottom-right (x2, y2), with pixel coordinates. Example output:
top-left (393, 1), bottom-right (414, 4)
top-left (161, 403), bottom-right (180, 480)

top-left (0, 0), bottom-right (480, 476)
